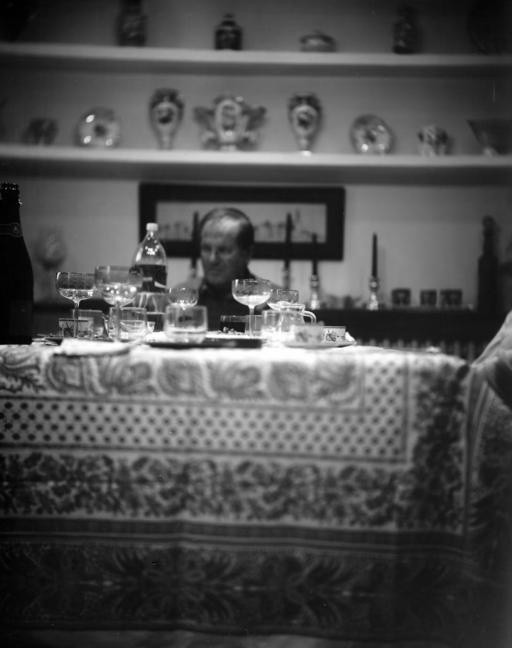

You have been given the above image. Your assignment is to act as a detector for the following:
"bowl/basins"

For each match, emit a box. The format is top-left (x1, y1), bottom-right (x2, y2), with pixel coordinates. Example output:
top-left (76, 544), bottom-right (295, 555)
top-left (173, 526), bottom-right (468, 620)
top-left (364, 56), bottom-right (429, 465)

top-left (466, 117), bottom-right (512, 157)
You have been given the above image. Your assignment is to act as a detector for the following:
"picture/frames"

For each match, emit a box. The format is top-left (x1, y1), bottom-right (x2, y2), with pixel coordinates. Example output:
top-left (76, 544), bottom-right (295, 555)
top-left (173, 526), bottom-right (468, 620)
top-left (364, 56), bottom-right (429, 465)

top-left (137, 179), bottom-right (343, 259)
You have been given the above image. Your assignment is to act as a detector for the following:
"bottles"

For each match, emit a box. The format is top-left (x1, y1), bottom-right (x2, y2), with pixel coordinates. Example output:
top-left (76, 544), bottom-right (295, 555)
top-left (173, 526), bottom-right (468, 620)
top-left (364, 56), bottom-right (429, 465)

top-left (0, 180), bottom-right (34, 346)
top-left (131, 221), bottom-right (167, 333)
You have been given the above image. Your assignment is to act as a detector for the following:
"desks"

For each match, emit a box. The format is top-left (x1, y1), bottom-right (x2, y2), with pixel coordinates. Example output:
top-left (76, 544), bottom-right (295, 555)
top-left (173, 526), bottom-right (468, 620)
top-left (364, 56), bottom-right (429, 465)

top-left (0, 347), bottom-right (512, 648)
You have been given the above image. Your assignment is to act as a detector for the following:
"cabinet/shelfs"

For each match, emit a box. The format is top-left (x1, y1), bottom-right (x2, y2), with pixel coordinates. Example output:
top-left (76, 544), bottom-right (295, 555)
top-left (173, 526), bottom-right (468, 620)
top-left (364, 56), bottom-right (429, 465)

top-left (0, 44), bottom-right (512, 183)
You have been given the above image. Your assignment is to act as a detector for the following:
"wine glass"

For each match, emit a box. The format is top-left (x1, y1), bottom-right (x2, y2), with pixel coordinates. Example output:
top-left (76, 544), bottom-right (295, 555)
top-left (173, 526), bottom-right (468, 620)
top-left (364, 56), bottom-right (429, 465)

top-left (57, 267), bottom-right (315, 345)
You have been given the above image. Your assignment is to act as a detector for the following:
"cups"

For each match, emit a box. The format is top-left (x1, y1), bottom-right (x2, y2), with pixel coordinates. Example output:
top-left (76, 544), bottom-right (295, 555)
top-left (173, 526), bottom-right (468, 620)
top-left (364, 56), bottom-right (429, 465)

top-left (391, 286), bottom-right (412, 308)
top-left (420, 289), bottom-right (437, 305)
top-left (441, 288), bottom-right (462, 308)
top-left (323, 326), bottom-right (346, 342)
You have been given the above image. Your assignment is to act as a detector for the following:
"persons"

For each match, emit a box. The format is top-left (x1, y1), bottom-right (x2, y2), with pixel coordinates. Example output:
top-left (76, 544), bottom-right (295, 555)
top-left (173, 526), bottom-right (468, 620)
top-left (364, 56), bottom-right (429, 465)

top-left (169, 207), bottom-right (282, 332)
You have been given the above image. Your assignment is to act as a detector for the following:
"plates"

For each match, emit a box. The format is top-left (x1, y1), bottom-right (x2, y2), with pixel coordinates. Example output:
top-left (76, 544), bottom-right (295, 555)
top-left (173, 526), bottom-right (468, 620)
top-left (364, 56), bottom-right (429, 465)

top-left (349, 114), bottom-right (393, 153)
top-left (286, 340), bottom-right (348, 350)
top-left (79, 107), bottom-right (121, 148)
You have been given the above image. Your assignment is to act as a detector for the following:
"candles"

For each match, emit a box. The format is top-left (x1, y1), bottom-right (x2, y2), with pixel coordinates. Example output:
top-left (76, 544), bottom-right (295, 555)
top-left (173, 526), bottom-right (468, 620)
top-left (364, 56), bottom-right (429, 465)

top-left (282, 212), bottom-right (293, 269)
top-left (310, 232), bottom-right (319, 273)
top-left (190, 209), bottom-right (201, 268)
top-left (371, 231), bottom-right (379, 277)
